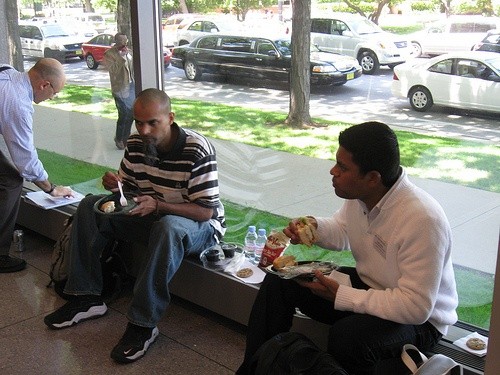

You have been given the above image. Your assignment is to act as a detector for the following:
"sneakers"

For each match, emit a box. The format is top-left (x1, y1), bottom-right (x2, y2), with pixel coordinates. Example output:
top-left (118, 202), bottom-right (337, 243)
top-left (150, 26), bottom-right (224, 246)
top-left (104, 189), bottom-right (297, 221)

top-left (44, 295), bottom-right (107, 329)
top-left (111, 321), bottom-right (160, 364)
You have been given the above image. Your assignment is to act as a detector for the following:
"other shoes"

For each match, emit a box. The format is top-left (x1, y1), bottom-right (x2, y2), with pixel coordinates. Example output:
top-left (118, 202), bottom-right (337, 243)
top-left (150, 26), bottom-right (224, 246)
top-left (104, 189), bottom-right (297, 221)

top-left (0, 255), bottom-right (26, 272)
top-left (114, 137), bottom-right (127, 150)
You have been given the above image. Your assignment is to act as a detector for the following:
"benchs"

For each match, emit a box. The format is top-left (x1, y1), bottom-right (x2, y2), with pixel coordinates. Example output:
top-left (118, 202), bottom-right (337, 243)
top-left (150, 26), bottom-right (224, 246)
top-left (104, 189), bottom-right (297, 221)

top-left (19, 180), bottom-right (489, 375)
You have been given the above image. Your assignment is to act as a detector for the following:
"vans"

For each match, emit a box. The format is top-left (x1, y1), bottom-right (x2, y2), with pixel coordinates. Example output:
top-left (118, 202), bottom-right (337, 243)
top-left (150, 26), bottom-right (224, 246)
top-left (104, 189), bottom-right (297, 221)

top-left (16, 10), bottom-right (107, 63)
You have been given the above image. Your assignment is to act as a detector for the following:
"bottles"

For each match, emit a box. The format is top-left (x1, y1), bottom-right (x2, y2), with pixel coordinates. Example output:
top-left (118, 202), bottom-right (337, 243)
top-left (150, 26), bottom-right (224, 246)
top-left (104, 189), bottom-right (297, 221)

top-left (254, 229), bottom-right (267, 264)
top-left (244, 227), bottom-right (257, 261)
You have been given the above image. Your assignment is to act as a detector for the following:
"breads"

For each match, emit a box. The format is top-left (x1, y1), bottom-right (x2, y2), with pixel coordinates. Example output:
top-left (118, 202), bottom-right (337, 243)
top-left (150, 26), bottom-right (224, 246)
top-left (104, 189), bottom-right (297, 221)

top-left (273, 255), bottom-right (296, 269)
top-left (101, 200), bottom-right (116, 213)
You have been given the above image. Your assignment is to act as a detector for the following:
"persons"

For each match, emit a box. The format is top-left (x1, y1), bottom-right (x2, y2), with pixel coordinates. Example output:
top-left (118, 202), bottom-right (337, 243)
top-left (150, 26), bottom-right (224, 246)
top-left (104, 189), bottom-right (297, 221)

top-left (235, 121), bottom-right (458, 375)
top-left (0, 58), bottom-right (76, 273)
top-left (44, 88), bottom-right (227, 363)
top-left (104, 32), bottom-right (135, 149)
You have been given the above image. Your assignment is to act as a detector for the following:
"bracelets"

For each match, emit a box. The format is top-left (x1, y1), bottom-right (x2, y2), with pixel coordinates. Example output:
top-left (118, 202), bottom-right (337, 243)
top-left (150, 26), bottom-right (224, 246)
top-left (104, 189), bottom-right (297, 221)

top-left (43, 183), bottom-right (55, 193)
top-left (153, 197), bottom-right (159, 216)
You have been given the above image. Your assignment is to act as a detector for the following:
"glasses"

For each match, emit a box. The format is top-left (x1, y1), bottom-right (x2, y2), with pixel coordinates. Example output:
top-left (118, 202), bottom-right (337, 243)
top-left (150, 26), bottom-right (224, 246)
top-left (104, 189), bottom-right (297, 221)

top-left (49, 83), bottom-right (59, 96)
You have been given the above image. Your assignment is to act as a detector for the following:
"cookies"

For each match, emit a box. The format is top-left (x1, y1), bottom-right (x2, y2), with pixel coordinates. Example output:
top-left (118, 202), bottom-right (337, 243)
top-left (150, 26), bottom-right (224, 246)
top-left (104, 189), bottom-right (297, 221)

top-left (237, 268), bottom-right (253, 277)
top-left (466, 337), bottom-right (486, 350)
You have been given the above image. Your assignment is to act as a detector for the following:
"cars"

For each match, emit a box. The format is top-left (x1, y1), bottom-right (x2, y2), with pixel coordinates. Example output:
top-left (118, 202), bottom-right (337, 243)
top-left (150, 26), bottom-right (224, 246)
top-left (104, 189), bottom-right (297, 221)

top-left (81, 33), bottom-right (172, 71)
top-left (160, 13), bottom-right (291, 49)
top-left (284, 12), bottom-right (412, 75)
top-left (392, 51), bottom-right (500, 116)
top-left (170, 33), bottom-right (363, 93)
top-left (406, 17), bottom-right (500, 59)
top-left (472, 31), bottom-right (500, 54)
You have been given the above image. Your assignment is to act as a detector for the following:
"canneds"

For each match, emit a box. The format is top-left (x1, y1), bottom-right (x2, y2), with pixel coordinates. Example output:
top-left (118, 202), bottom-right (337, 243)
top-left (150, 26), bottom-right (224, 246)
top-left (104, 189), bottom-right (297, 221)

top-left (13, 229), bottom-right (25, 251)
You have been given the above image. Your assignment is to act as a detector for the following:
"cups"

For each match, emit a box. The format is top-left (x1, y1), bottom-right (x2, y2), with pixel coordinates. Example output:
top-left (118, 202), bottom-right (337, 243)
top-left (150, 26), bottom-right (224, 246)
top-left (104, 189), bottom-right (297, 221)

top-left (222, 243), bottom-right (237, 257)
top-left (204, 250), bottom-right (220, 265)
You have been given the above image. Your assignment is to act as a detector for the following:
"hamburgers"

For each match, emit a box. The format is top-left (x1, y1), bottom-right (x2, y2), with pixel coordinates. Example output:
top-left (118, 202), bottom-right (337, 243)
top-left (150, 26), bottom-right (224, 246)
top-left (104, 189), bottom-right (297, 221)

top-left (296, 216), bottom-right (323, 249)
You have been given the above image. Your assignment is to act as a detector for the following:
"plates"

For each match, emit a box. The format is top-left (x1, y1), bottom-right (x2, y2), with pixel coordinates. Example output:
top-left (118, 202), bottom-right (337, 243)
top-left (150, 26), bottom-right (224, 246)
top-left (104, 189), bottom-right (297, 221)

top-left (94, 192), bottom-right (139, 214)
top-left (265, 260), bottom-right (338, 278)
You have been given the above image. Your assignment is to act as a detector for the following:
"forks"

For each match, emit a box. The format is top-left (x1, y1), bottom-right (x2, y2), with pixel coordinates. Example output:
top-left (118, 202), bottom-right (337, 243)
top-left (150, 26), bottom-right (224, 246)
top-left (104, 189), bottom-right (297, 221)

top-left (117, 181), bottom-right (128, 206)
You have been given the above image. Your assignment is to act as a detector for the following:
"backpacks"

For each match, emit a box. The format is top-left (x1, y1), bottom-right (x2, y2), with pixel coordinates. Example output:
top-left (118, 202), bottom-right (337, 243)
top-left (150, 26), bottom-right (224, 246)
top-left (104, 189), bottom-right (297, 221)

top-left (45, 214), bottom-right (129, 302)
top-left (247, 332), bottom-right (349, 375)
top-left (401, 344), bottom-right (463, 375)
